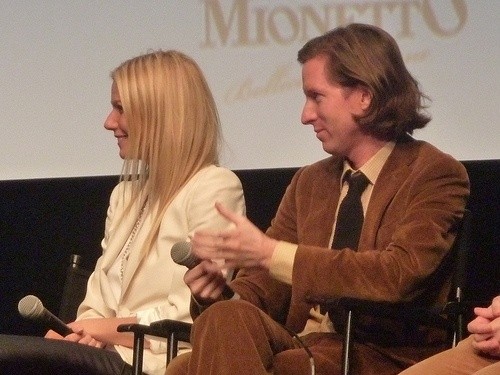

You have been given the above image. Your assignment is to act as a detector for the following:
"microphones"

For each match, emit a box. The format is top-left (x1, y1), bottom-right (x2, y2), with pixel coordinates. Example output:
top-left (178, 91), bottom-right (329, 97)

top-left (171, 241), bottom-right (241, 300)
top-left (18, 294), bottom-right (75, 337)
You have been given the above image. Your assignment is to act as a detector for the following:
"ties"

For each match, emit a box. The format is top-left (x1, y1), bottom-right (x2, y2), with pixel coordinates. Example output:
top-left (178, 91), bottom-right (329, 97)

top-left (327, 170), bottom-right (371, 316)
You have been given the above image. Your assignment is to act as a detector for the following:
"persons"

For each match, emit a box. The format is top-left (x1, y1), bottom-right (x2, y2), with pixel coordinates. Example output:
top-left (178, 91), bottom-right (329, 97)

top-left (165, 22), bottom-right (473, 375)
top-left (0, 48), bottom-right (248, 375)
top-left (467, 296), bottom-right (500, 362)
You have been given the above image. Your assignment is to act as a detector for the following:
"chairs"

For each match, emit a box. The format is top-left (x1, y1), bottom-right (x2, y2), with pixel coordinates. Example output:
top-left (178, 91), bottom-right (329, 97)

top-left (57, 207), bottom-right (500, 375)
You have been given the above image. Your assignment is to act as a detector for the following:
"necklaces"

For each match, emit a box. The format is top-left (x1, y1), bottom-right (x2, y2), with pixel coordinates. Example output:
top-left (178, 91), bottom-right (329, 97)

top-left (120, 199), bottom-right (149, 287)
